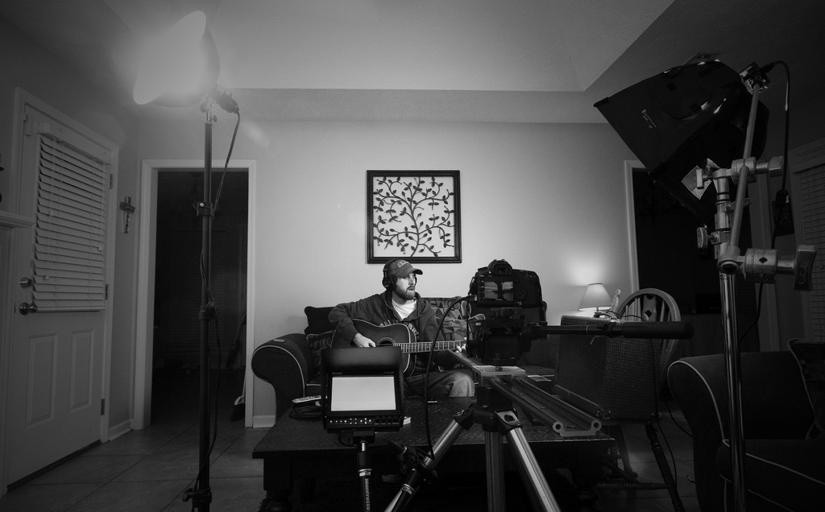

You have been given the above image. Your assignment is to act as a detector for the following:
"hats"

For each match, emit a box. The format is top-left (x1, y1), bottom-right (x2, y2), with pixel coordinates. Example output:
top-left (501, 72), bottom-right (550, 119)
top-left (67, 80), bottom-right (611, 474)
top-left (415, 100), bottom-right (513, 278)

top-left (383, 257), bottom-right (425, 277)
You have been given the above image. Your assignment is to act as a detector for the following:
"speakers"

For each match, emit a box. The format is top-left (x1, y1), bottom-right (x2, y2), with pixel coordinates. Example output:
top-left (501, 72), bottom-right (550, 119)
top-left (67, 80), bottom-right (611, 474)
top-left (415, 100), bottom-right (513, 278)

top-left (561, 318), bottom-right (694, 414)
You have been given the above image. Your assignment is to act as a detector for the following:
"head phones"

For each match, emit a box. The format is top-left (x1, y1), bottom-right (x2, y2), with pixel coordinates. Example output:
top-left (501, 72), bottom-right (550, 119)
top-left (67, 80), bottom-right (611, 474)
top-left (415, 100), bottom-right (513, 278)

top-left (381, 259), bottom-right (410, 291)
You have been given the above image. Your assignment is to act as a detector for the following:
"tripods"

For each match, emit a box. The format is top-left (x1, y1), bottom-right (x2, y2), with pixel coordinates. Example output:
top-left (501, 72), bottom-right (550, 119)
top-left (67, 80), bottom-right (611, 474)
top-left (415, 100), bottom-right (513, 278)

top-left (382, 408), bottom-right (561, 512)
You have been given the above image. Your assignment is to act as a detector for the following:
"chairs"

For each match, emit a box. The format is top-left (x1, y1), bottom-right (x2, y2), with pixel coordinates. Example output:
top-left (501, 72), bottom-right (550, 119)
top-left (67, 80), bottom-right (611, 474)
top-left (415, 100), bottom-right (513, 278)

top-left (667, 349), bottom-right (823, 506)
top-left (616, 287), bottom-right (683, 378)
top-left (251, 299), bottom-right (472, 421)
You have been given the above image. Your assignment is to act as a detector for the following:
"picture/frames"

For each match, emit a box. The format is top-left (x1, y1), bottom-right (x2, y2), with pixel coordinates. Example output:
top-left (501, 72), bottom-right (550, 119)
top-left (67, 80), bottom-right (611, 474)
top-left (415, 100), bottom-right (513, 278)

top-left (366, 169), bottom-right (460, 265)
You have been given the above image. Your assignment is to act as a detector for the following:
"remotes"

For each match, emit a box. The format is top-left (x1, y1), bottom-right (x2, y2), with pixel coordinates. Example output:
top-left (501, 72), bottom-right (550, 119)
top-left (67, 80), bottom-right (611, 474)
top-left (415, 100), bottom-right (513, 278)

top-left (293, 394), bottom-right (321, 403)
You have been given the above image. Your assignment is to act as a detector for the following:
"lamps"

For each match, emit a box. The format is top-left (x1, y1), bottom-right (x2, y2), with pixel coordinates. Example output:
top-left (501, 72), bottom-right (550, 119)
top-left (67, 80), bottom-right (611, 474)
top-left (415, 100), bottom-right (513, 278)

top-left (130, 8), bottom-right (242, 512)
top-left (578, 283), bottom-right (612, 318)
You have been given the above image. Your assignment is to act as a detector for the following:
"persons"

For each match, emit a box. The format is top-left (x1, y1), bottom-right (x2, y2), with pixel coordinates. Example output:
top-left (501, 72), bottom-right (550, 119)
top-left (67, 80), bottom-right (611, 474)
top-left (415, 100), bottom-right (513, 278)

top-left (327, 258), bottom-right (476, 397)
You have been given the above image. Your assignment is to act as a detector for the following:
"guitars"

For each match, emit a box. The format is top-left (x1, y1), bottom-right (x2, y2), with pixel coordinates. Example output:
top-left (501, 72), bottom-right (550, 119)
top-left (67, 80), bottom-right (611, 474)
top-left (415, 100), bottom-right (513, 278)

top-left (329, 323), bottom-right (469, 375)
